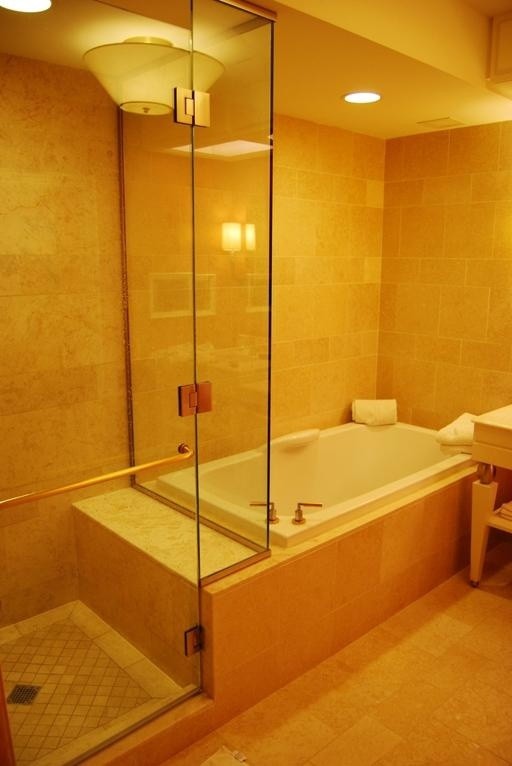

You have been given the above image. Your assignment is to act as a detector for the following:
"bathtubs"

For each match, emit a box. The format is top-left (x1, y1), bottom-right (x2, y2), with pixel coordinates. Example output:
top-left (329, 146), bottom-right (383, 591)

top-left (156, 422), bottom-right (477, 549)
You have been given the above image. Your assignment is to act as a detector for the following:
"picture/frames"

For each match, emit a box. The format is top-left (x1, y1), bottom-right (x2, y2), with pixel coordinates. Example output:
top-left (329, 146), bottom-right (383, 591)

top-left (147, 271), bottom-right (217, 319)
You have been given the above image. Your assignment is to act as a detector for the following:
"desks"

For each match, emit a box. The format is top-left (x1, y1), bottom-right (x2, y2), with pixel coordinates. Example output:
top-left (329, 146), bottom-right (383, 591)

top-left (469, 405), bottom-right (512, 587)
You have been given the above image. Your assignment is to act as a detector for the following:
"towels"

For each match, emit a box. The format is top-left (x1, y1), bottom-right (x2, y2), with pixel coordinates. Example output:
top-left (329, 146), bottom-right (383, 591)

top-left (494, 499), bottom-right (512, 521)
top-left (351, 399), bottom-right (398, 428)
top-left (436, 411), bottom-right (477, 452)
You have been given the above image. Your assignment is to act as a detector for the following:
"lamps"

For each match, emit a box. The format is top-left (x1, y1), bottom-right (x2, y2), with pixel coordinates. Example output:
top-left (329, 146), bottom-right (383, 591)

top-left (220, 220), bottom-right (256, 265)
top-left (81, 36), bottom-right (224, 115)
